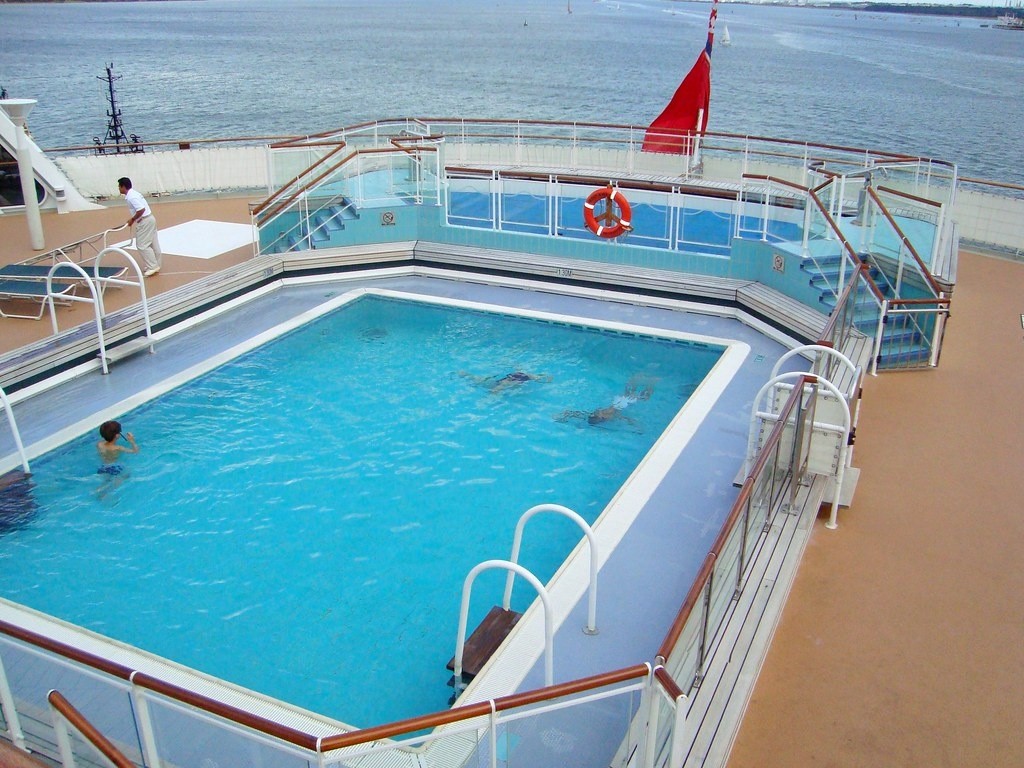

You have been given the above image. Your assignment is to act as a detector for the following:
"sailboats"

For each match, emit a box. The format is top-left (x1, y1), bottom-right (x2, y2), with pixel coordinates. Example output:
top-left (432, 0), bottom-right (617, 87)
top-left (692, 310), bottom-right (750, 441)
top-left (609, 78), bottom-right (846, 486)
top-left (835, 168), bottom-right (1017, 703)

top-left (568, 0), bottom-right (572, 14)
top-left (719, 24), bottom-right (731, 46)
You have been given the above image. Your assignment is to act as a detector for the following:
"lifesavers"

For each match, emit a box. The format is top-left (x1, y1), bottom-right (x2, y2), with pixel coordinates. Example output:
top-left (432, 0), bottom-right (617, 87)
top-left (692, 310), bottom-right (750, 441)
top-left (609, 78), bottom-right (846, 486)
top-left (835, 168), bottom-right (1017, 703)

top-left (583, 188), bottom-right (631, 238)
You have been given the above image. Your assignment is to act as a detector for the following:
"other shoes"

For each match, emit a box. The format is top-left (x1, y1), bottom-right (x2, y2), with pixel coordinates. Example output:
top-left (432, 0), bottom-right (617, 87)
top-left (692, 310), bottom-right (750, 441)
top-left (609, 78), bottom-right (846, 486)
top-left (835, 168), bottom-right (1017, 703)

top-left (144, 266), bottom-right (161, 276)
top-left (143, 266), bottom-right (160, 273)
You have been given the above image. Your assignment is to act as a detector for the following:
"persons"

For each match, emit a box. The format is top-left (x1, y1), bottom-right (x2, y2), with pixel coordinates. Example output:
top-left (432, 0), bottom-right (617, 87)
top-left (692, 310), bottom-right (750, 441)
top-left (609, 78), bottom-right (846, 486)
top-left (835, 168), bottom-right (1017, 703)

top-left (99, 421), bottom-right (133, 444)
top-left (118, 177), bottom-right (161, 276)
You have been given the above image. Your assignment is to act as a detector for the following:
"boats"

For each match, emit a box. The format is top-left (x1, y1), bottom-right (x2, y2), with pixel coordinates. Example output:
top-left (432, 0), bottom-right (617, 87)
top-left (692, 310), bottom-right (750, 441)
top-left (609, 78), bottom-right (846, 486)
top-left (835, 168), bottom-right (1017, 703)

top-left (997, 10), bottom-right (1022, 26)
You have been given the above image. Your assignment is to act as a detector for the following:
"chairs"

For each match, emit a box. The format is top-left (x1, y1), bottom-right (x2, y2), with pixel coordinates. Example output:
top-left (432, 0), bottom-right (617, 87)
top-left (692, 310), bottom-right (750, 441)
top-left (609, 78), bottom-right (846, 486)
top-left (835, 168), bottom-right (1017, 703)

top-left (0, 264), bottom-right (128, 320)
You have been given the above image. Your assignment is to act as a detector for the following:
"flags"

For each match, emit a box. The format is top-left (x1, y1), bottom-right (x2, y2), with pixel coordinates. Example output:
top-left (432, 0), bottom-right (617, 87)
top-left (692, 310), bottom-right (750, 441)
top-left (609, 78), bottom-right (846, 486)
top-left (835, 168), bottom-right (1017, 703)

top-left (641, 0), bottom-right (718, 157)
